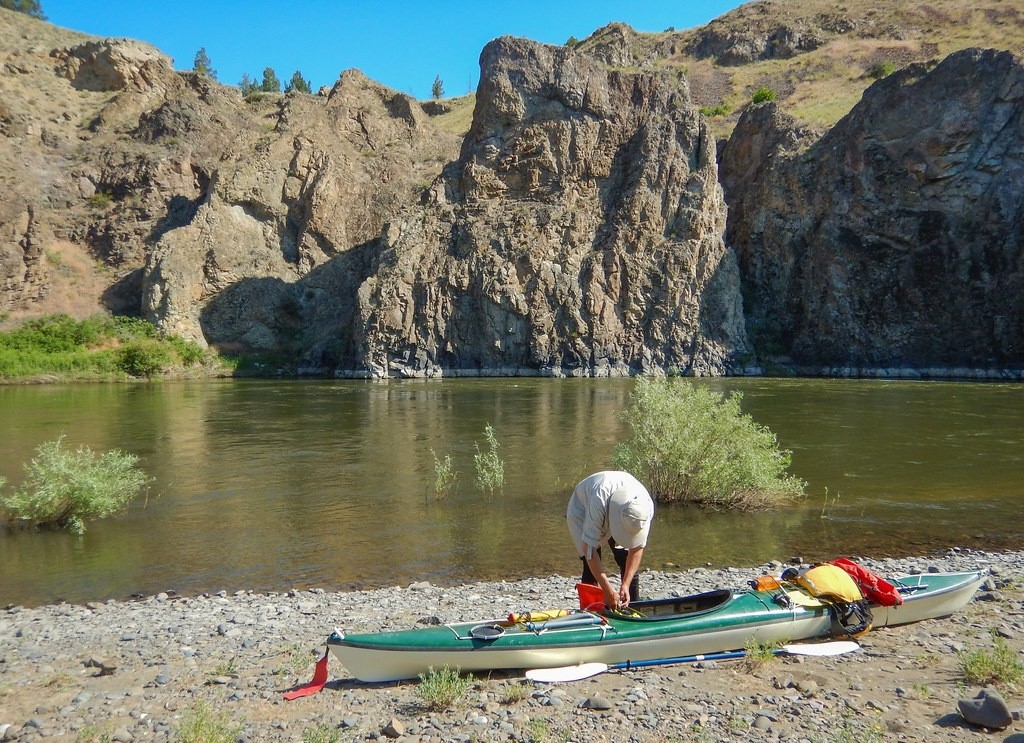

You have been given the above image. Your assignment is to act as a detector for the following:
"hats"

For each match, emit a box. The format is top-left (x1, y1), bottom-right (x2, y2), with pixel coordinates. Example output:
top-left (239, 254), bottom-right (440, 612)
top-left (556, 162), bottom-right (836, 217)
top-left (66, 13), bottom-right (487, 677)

top-left (608, 488), bottom-right (652, 548)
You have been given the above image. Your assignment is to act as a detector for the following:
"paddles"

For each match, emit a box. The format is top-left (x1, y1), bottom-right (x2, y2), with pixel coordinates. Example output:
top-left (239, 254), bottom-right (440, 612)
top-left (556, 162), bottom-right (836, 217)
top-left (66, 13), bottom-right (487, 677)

top-left (526, 642), bottom-right (862, 682)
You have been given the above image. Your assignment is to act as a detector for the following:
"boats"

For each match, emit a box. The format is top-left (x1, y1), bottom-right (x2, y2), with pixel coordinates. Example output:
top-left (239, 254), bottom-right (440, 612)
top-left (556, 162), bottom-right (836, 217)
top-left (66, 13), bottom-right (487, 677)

top-left (280, 571), bottom-right (999, 701)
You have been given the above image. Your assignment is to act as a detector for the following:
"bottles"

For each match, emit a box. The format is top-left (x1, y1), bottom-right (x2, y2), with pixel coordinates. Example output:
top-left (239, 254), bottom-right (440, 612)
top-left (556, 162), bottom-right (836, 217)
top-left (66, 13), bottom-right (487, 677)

top-left (746, 576), bottom-right (799, 593)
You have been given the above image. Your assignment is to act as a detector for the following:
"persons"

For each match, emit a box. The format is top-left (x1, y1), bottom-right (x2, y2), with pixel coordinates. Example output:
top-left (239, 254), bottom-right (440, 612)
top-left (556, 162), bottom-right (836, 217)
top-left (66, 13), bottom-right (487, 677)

top-left (566, 470), bottom-right (656, 612)
